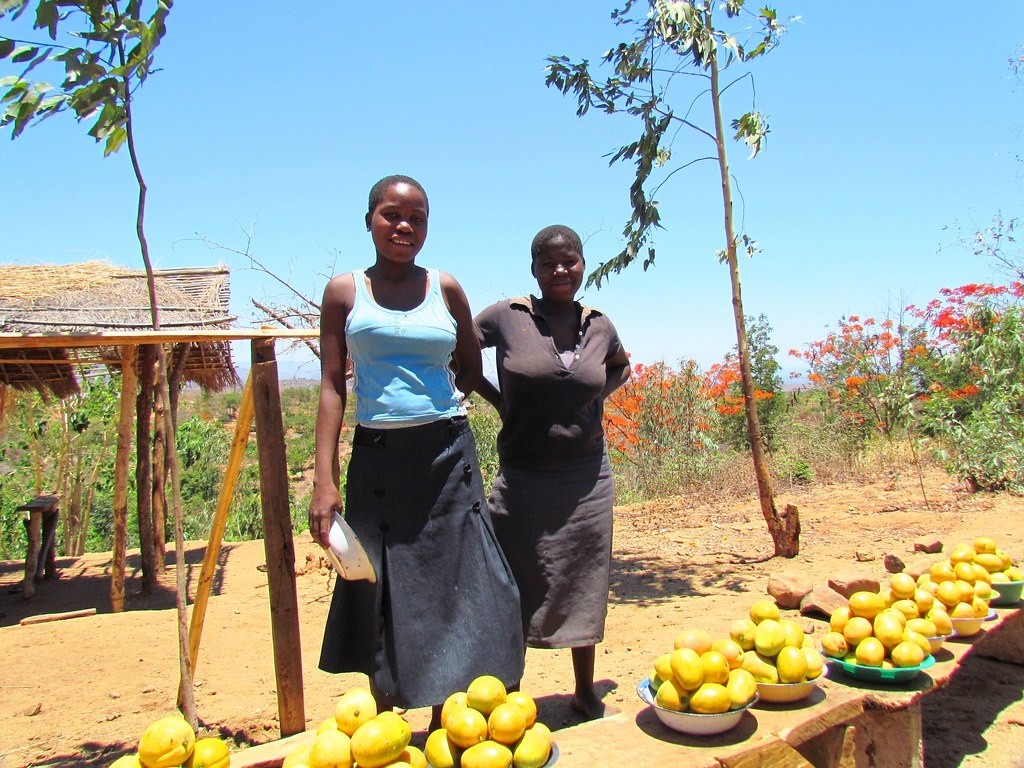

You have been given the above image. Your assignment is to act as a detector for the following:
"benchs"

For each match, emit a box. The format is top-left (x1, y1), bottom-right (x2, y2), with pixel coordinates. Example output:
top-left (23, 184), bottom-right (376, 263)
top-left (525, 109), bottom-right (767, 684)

top-left (16, 492), bottom-right (63, 598)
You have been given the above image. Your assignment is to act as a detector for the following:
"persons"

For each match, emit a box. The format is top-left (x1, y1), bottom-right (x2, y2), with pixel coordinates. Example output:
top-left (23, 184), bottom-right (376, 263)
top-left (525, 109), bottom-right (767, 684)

top-left (308, 175), bottom-right (525, 735)
top-left (472, 225), bottom-right (630, 717)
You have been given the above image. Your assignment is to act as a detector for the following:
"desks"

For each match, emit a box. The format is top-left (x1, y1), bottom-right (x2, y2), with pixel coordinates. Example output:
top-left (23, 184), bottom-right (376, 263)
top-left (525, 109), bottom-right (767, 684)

top-left (229, 561), bottom-right (1024, 768)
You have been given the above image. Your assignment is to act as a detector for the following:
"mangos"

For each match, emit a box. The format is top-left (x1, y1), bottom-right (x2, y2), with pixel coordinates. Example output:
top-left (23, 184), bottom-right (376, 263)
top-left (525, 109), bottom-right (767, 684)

top-left (108, 717), bottom-right (230, 768)
top-left (281, 676), bottom-right (553, 768)
top-left (647, 538), bottom-right (1023, 714)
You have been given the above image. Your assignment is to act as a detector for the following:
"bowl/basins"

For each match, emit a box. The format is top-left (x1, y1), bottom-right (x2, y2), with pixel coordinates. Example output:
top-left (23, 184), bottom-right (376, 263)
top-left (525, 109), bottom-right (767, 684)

top-left (982, 589), bottom-right (1001, 607)
top-left (991, 580), bottom-right (1024, 607)
top-left (819, 647), bottom-right (936, 685)
top-left (927, 637), bottom-right (945, 656)
top-left (950, 608), bottom-right (998, 637)
top-left (636, 677), bottom-right (760, 735)
top-left (320, 512), bottom-right (376, 583)
top-left (757, 662), bottom-right (829, 703)
top-left (422, 741), bottom-right (560, 768)
